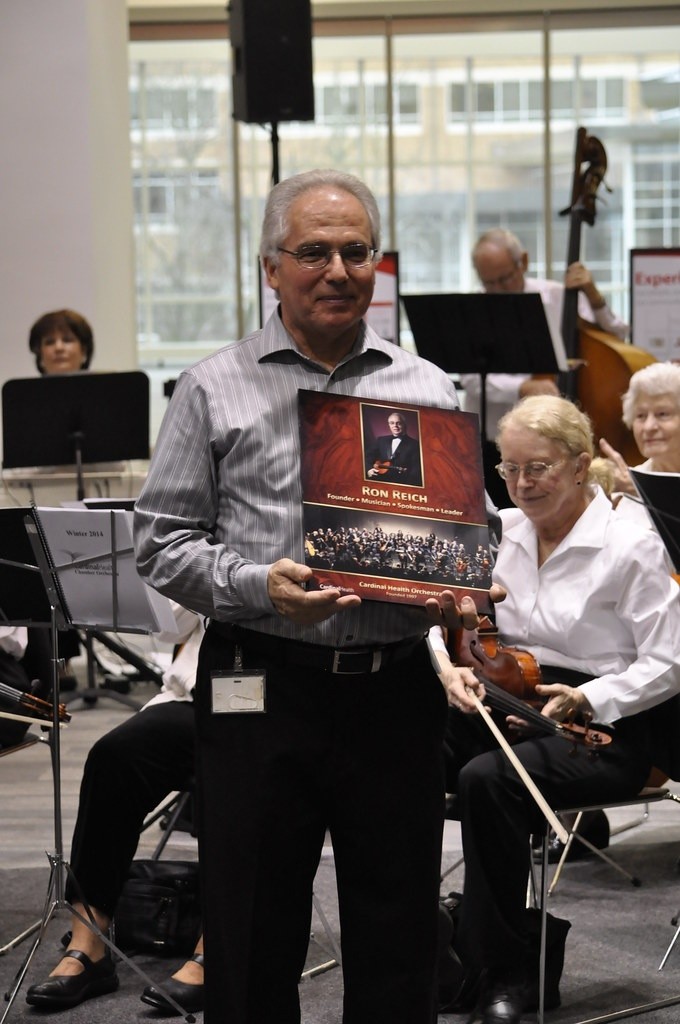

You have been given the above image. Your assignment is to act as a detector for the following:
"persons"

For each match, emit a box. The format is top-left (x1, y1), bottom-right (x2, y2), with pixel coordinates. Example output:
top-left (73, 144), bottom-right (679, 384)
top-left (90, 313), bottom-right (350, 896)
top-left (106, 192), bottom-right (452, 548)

top-left (304, 523), bottom-right (492, 589)
top-left (29, 612), bottom-right (210, 1013)
top-left (451, 232), bottom-right (680, 1024)
top-left (0, 309), bottom-right (138, 688)
top-left (363, 412), bottom-right (424, 485)
top-left (135, 164), bottom-right (508, 1024)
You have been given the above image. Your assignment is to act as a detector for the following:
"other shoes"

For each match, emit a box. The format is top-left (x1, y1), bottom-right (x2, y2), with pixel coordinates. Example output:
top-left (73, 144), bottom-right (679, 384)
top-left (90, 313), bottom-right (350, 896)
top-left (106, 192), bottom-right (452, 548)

top-left (60, 666), bottom-right (77, 691)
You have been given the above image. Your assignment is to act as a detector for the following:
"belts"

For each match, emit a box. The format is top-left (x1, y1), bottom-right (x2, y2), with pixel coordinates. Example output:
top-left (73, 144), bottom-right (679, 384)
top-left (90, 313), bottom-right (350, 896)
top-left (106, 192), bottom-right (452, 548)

top-left (207, 616), bottom-right (422, 676)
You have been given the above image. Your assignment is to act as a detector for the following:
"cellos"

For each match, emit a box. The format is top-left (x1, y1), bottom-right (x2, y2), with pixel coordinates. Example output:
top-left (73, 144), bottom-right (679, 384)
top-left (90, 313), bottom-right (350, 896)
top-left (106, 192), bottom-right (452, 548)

top-left (530, 128), bottom-right (662, 467)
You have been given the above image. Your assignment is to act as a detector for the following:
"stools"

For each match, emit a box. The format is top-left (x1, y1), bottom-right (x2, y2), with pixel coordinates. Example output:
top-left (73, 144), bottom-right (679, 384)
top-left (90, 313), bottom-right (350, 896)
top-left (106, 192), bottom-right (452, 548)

top-left (443, 784), bottom-right (678, 1023)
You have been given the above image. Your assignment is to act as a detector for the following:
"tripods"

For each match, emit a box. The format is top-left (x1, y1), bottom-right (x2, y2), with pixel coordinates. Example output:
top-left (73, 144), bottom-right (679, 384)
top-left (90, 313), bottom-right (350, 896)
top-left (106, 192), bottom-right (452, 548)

top-left (0, 506), bottom-right (197, 1024)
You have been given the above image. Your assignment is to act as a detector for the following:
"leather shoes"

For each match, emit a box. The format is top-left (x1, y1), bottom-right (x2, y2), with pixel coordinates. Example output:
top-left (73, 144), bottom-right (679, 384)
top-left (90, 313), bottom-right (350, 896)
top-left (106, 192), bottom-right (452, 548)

top-left (436, 906), bottom-right (462, 985)
top-left (532, 810), bottom-right (609, 864)
top-left (25, 950), bottom-right (119, 1006)
top-left (485, 979), bottom-right (532, 1024)
top-left (141, 953), bottom-right (204, 1013)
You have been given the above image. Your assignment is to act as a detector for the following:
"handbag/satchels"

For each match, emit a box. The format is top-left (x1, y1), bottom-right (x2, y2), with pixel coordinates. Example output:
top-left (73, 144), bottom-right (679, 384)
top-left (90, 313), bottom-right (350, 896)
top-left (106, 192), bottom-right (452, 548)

top-left (114, 860), bottom-right (202, 958)
top-left (432, 893), bottom-right (571, 1012)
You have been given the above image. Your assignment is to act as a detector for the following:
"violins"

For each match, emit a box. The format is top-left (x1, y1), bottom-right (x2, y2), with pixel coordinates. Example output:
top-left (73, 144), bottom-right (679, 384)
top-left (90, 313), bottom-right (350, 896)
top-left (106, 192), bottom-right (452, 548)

top-left (372, 460), bottom-right (408, 475)
top-left (446, 614), bottom-right (612, 780)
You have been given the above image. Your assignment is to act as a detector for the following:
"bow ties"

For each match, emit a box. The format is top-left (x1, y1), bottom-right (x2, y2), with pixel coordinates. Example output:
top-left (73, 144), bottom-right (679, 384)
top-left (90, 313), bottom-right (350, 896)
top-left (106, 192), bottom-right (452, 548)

top-left (392, 435), bottom-right (402, 439)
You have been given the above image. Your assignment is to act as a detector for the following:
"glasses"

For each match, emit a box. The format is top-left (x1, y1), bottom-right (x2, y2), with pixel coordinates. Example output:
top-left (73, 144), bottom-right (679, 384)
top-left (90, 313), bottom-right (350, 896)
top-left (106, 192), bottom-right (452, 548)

top-left (277, 244), bottom-right (378, 268)
top-left (495, 459), bottom-right (562, 478)
top-left (482, 262), bottom-right (517, 285)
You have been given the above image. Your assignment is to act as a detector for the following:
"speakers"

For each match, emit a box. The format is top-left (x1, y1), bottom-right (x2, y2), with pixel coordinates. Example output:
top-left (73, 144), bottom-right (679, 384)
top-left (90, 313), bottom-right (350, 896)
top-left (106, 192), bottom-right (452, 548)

top-left (225, 0), bottom-right (314, 123)
